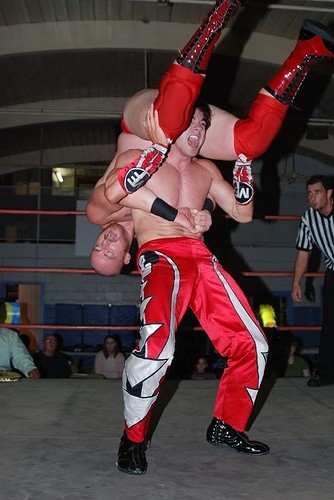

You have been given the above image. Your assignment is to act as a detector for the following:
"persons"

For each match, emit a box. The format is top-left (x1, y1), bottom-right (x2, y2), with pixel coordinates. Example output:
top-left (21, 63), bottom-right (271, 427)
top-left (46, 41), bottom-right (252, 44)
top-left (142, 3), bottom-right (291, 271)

top-left (86, 0), bottom-right (334, 278)
top-left (291, 175), bottom-right (334, 386)
top-left (283, 340), bottom-right (310, 377)
top-left (0, 328), bottom-right (41, 379)
top-left (206, 347), bottom-right (228, 378)
top-left (93, 335), bottom-right (125, 379)
top-left (189, 357), bottom-right (215, 379)
top-left (31, 334), bottom-right (72, 378)
top-left (104, 102), bottom-right (270, 474)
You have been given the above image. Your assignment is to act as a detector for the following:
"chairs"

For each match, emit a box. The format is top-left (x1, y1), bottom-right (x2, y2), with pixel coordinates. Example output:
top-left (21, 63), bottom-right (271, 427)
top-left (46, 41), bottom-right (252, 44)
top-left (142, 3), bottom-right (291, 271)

top-left (42, 300), bottom-right (321, 379)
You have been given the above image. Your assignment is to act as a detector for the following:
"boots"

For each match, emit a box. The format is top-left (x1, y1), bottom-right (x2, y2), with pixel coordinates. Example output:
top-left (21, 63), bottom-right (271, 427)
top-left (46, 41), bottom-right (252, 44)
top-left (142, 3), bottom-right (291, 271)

top-left (261, 19), bottom-right (334, 106)
top-left (174, 0), bottom-right (242, 74)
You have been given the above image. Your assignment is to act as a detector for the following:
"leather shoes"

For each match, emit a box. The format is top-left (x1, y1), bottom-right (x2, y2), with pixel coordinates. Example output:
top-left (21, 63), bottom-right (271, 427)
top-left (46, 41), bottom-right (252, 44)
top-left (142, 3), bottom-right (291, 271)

top-left (205, 416), bottom-right (269, 455)
top-left (116, 431), bottom-right (148, 475)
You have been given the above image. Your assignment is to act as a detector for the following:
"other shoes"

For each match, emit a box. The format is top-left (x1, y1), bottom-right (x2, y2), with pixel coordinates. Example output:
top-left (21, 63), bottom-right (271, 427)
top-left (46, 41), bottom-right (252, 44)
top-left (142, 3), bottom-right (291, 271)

top-left (306, 375), bottom-right (334, 387)
